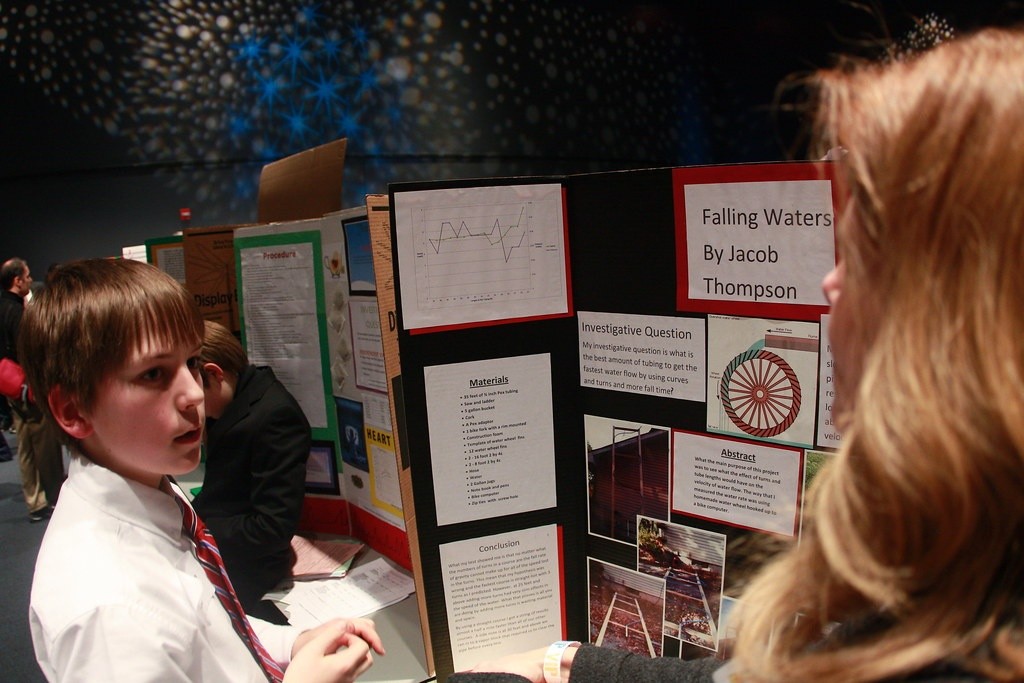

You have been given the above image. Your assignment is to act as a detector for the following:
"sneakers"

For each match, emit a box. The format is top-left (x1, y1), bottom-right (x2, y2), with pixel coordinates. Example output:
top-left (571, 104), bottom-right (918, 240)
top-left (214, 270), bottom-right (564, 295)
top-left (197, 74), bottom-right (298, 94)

top-left (24, 505), bottom-right (56, 522)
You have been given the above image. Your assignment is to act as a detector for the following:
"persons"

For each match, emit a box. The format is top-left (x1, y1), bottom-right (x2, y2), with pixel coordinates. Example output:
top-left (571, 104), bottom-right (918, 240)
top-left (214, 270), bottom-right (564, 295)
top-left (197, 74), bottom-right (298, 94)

top-left (27, 255), bottom-right (386, 682)
top-left (447, 34), bottom-right (1023, 682)
top-left (192, 318), bottom-right (312, 616)
top-left (0, 258), bottom-right (59, 523)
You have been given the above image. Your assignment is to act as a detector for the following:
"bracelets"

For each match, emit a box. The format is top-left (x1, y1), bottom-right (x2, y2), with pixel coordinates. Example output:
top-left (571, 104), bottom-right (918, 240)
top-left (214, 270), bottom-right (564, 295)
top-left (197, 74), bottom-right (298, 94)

top-left (543, 640), bottom-right (578, 683)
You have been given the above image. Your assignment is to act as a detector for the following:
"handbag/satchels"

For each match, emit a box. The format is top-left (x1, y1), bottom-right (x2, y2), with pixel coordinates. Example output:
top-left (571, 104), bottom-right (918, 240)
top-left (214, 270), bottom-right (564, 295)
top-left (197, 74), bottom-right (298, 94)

top-left (0, 356), bottom-right (50, 426)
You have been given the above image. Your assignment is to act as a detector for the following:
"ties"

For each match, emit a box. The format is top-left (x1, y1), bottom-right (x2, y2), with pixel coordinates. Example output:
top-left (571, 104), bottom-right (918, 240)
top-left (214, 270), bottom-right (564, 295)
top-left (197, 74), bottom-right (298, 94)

top-left (175, 495), bottom-right (286, 683)
